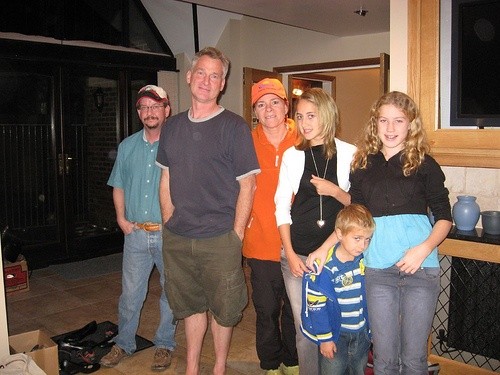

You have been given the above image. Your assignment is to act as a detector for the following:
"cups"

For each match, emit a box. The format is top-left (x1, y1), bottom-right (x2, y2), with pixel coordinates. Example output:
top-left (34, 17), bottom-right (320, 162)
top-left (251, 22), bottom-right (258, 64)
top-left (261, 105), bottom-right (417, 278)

top-left (480, 211), bottom-right (500, 235)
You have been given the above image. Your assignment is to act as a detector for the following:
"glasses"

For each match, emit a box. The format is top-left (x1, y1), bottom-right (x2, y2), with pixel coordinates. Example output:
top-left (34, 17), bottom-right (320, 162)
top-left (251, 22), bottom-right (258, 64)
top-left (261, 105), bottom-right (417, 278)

top-left (139, 104), bottom-right (165, 111)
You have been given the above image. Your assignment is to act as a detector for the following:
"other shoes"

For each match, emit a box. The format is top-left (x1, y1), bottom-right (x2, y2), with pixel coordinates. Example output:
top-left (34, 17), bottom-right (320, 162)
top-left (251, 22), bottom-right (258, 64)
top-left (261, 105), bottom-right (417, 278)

top-left (282, 363), bottom-right (299, 375)
top-left (267, 366), bottom-right (282, 375)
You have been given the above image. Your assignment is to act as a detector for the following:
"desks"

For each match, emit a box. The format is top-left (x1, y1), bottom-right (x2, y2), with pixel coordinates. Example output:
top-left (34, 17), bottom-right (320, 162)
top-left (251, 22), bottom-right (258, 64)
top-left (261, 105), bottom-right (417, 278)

top-left (425, 225), bottom-right (500, 375)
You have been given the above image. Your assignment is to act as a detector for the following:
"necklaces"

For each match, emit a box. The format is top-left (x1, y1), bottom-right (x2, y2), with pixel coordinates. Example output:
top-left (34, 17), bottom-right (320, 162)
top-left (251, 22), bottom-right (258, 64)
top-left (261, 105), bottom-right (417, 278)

top-left (309, 146), bottom-right (330, 228)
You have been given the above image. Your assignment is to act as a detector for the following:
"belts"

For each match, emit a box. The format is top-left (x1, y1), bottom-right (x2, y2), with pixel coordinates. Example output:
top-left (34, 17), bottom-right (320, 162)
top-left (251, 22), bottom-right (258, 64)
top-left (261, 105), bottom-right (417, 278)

top-left (130, 222), bottom-right (162, 232)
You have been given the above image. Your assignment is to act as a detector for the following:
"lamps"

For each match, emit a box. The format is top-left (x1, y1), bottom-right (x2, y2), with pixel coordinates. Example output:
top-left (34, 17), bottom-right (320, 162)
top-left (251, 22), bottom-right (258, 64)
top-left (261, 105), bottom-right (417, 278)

top-left (95, 87), bottom-right (104, 112)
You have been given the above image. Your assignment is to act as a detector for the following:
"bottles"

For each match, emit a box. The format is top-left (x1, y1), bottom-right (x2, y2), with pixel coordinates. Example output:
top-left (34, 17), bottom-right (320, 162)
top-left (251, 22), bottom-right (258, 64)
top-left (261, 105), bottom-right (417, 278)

top-left (452, 195), bottom-right (480, 231)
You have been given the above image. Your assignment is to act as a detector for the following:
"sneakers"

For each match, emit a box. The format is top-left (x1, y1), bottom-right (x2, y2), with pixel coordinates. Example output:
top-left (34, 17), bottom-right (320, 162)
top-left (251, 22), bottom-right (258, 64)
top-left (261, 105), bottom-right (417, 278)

top-left (101, 344), bottom-right (133, 367)
top-left (152, 348), bottom-right (172, 370)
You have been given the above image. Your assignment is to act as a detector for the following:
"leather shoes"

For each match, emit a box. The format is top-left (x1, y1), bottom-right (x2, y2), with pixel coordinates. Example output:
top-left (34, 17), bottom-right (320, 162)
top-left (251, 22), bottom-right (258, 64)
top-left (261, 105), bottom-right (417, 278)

top-left (59, 341), bottom-right (96, 352)
top-left (61, 360), bottom-right (100, 375)
top-left (99, 331), bottom-right (118, 347)
top-left (64, 321), bottom-right (97, 342)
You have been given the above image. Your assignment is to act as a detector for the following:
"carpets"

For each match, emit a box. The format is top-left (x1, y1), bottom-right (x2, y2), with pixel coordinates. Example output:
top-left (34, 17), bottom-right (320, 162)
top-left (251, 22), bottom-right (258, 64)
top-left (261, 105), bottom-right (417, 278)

top-left (51, 320), bottom-right (155, 375)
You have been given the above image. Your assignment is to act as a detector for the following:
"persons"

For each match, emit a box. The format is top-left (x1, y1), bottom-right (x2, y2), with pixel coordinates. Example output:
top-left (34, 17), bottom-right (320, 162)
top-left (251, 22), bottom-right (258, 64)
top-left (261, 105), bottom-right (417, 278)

top-left (274, 87), bottom-right (363, 374)
top-left (155, 45), bottom-right (261, 374)
top-left (239, 79), bottom-right (307, 374)
top-left (306, 92), bottom-right (453, 375)
top-left (299, 203), bottom-right (375, 374)
top-left (99, 86), bottom-right (180, 373)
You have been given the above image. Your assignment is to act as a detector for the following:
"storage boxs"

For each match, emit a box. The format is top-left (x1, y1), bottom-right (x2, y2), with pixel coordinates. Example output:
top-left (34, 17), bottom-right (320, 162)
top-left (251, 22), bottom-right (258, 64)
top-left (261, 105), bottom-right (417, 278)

top-left (8, 329), bottom-right (59, 375)
top-left (3, 254), bottom-right (30, 294)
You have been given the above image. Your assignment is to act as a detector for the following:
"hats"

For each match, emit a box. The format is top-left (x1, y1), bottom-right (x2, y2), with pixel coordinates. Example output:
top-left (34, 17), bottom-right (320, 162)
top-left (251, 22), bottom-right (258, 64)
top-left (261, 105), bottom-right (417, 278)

top-left (252, 78), bottom-right (288, 108)
top-left (137, 85), bottom-right (168, 106)
